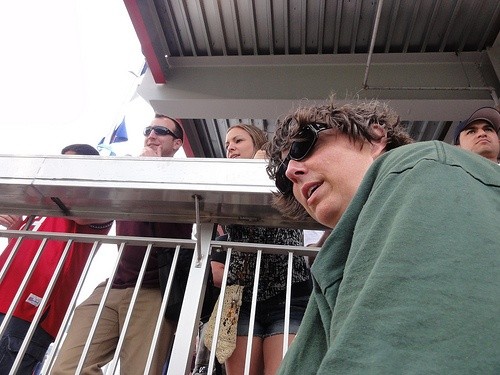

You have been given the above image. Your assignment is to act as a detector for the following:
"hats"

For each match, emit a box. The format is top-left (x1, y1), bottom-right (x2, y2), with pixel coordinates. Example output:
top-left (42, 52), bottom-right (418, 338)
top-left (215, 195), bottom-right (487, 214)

top-left (453, 106), bottom-right (500, 143)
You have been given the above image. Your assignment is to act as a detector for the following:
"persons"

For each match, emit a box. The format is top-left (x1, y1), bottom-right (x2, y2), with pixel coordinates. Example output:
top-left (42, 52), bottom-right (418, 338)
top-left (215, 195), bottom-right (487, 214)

top-left (224, 126), bottom-right (311, 375)
top-left (0, 144), bottom-right (113, 375)
top-left (191, 233), bottom-right (229, 375)
top-left (453, 106), bottom-right (500, 166)
top-left (261, 89), bottom-right (500, 375)
top-left (50, 113), bottom-right (194, 375)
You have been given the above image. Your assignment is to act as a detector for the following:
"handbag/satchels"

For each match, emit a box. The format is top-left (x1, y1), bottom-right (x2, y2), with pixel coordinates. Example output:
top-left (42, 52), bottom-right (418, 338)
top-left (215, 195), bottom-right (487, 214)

top-left (200, 281), bottom-right (247, 364)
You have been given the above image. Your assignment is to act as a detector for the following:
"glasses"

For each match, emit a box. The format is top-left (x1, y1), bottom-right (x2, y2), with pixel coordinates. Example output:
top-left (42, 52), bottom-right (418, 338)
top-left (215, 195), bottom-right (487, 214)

top-left (274, 124), bottom-right (339, 194)
top-left (143, 125), bottom-right (177, 139)
